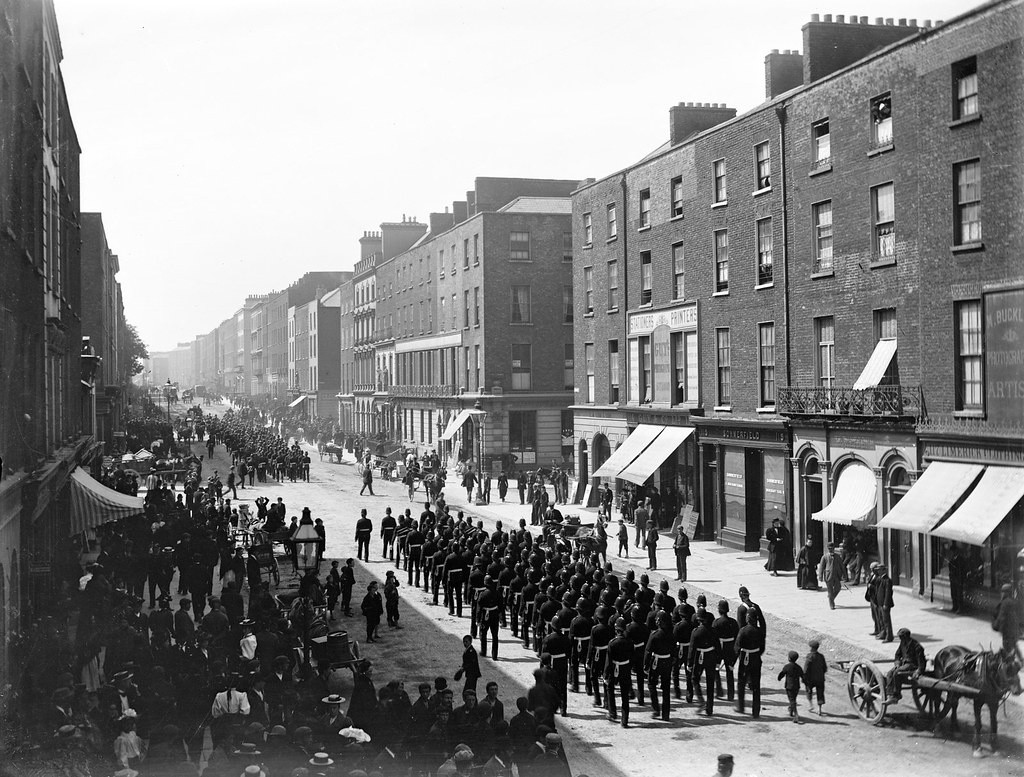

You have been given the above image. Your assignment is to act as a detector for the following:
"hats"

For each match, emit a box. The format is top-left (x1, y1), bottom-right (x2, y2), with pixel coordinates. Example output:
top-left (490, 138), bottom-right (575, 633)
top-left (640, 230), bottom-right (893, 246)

top-left (809, 640), bottom-right (820, 647)
top-left (162, 547), bottom-right (175, 553)
top-left (773, 516), bottom-right (780, 522)
top-left (789, 651), bottom-right (800, 660)
top-left (52, 687), bottom-right (73, 700)
top-left (492, 735), bottom-right (513, 750)
top-left (478, 701), bottom-right (492, 716)
top-left (309, 752), bottom-right (334, 766)
top-left (275, 655), bottom-right (290, 662)
top-left (874, 562), bottom-right (888, 569)
top-left (74, 682), bottom-right (88, 692)
top-left (111, 670), bottom-right (133, 682)
top-left (240, 764), bottom-right (266, 777)
top-left (897, 626), bottom-right (912, 638)
top-left (827, 541), bottom-right (833, 548)
top-left (269, 725), bottom-right (286, 736)
top-left (322, 694), bottom-right (346, 703)
top-left (234, 742), bottom-right (262, 754)
top-left (292, 726), bottom-right (311, 738)
top-left (545, 733), bottom-right (563, 744)
top-left (868, 562), bottom-right (878, 569)
top-left (677, 526), bottom-right (685, 532)
top-left (238, 618), bottom-right (256, 626)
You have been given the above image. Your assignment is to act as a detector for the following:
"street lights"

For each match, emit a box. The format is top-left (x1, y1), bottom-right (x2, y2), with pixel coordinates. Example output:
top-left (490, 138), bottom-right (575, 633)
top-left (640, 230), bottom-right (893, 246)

top-left (468, 400), bottom-right (489, 506)
top-left (289, 507), bottom-right (325, 698)
top-left (164, 377), bottom-right (173, 423)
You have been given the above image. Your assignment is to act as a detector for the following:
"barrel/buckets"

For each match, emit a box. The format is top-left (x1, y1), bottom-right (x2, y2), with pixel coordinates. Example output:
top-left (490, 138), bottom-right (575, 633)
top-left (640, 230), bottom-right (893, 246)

top-left (326, 630), bottom-right (352, 663)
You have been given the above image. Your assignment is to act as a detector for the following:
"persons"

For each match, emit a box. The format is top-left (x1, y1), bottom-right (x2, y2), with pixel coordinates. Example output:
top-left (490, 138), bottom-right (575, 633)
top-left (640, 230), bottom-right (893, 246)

top-left (0, 381), bottom-right (1024, 777)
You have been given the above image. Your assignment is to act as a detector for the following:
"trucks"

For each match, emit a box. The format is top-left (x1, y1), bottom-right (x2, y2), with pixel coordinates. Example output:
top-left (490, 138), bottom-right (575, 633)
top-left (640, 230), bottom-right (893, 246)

top-left (195, 386), bottom-right (206, 396)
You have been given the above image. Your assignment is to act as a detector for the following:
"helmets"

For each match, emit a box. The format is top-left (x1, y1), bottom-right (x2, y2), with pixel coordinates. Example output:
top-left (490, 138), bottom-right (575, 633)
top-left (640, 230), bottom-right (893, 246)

top-left (361, 502), bottom-right (760, 632)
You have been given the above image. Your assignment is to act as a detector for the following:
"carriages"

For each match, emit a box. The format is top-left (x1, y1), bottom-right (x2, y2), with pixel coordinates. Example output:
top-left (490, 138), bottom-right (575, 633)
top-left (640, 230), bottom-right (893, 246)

top-left (829, 638), bottom-right (1023, 760)
top-left (369, 454), bottom-right (397, 482)
top-left (119, 448), bottom-right (204, 488)
top-left (316, 433), bottom-right (343, 463)
top-left (228, 504), bottom-right (360, 689)
top-left (550, 510), bottom-right (609, 568)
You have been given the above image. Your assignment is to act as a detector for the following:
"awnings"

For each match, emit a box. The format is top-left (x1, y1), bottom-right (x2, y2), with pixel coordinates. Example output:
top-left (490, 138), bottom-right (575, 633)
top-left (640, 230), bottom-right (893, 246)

top-left (927, 466), bottom-right (1024, 547)
top-left (69, 466), bottom-right (144, 538)
top-left (812, 464), bottom-right (878, 525)
top-left (591, 424), bottom-right (666, 477)
top-left (875, 461), bottom-right (985, 533)
top-left (439, 411), bottom-right (470, 440)
top-left (616, 426), bottom-right (695, 487)
top-left (288, 396), bottom-right (307, 407)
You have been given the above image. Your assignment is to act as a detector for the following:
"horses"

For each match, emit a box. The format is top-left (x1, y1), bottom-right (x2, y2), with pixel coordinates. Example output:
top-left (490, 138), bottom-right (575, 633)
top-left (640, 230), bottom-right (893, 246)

top-left (574, 510), bottom-right (608, 568)
top-left (245, 511), bottom-right (267, 547)
top-left (276, 592), bottom-right (303, 609)
top-left (156, 454), bottom-right (201, 484)
top-left (934, 644), bottom-right (1024, 759)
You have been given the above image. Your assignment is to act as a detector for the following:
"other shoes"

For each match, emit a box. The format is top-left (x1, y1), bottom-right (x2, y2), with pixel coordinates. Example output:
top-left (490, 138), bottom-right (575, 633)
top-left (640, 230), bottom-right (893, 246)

top-left (510, 632), bottom-right (825, 729)
top-left (881, 694), bottom-right (901, 707)
top-left (357, 553), bottom-right (826, 723)
